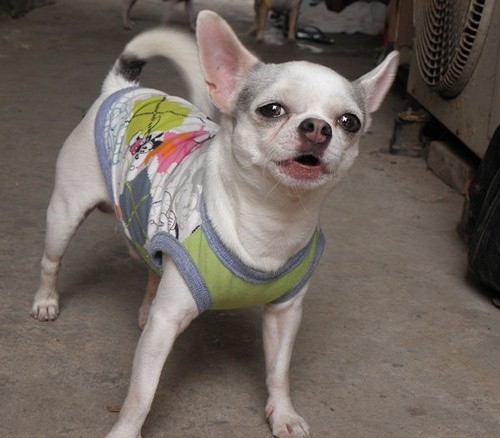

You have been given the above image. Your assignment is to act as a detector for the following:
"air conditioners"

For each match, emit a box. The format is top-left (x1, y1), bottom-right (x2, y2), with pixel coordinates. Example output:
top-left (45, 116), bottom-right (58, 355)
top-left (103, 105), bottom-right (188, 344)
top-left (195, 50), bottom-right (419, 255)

top-left (404, 0), bottom-right (500, 158)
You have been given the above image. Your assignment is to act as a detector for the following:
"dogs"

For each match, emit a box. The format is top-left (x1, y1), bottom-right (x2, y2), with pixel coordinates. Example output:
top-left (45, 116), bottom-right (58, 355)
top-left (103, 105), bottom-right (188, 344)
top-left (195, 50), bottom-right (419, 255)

top-left (31, 9), bottom-right (398, 436)
